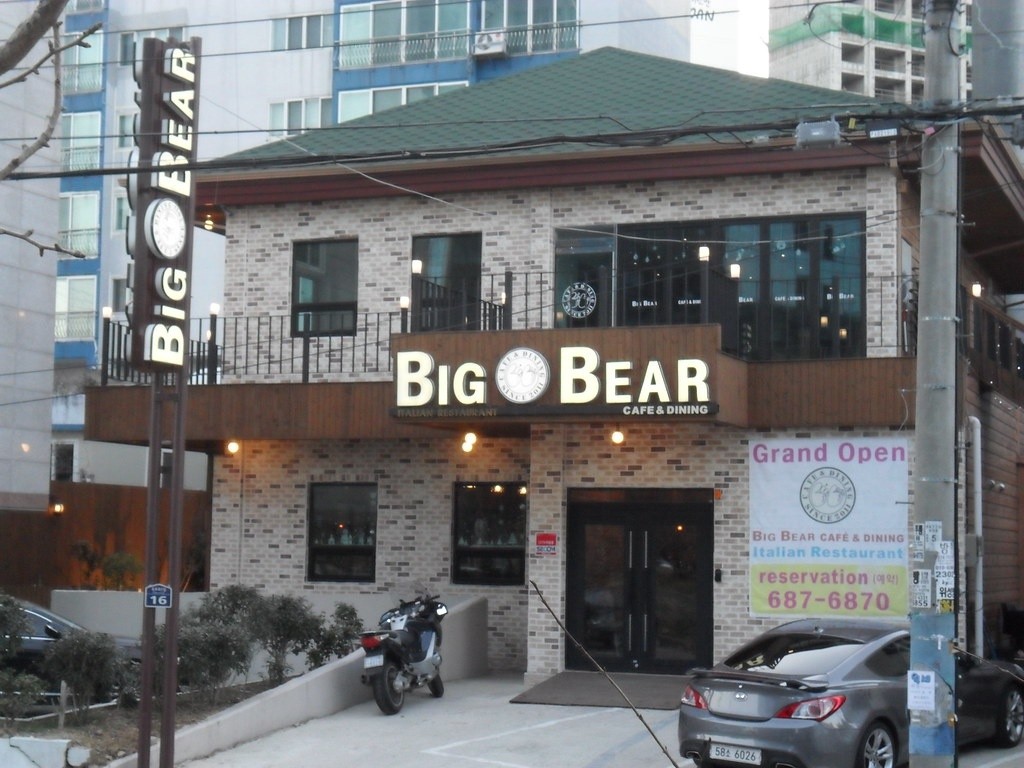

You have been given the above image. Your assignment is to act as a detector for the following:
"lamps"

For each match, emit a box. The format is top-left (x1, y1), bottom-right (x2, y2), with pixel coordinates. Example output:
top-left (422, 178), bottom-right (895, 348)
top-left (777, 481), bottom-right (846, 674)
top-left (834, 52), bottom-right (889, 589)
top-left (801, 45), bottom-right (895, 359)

top-left (730, 263), bottom-right (741, 278)
top-left (103, 306), bottom-right (112, 319)
top-left (411, 259), bottom-right (423, 274)
top-left (399, 296), bottom-right (410, 308)
top-left (209, 302), bottom-right (220, 315)
top-left (204, 214), bottom-right (213, 230)
top-left (699, 245), bottom-right (709, 261)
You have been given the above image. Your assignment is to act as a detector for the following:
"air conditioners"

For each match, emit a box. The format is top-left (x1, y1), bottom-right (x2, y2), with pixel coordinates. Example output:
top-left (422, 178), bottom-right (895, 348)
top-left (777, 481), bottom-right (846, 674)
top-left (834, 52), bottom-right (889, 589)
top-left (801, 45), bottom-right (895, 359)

top-left (473, 30), bottom-right (508, 58)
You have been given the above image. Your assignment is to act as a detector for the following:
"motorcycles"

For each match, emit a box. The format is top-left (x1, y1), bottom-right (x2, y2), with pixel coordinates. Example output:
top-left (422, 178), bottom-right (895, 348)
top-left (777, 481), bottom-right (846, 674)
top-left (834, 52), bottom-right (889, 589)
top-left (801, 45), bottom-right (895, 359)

top-left (358, 580), bottom-right (449, 716)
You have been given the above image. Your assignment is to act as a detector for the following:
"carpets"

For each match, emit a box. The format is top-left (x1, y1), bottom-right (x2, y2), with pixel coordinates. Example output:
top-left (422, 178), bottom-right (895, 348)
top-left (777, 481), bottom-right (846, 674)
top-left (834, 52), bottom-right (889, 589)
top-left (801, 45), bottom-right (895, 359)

top-left (508, 672), bottom-right (694, 711)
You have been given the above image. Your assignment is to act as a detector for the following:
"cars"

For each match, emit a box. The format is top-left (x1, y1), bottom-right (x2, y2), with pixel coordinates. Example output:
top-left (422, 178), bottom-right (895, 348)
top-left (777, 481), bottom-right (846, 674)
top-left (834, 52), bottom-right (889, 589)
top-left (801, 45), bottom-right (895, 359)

top-left (679, 616), bottom-right (1023, 768)
top-left (0, 596), bottom-right (144, 715)
